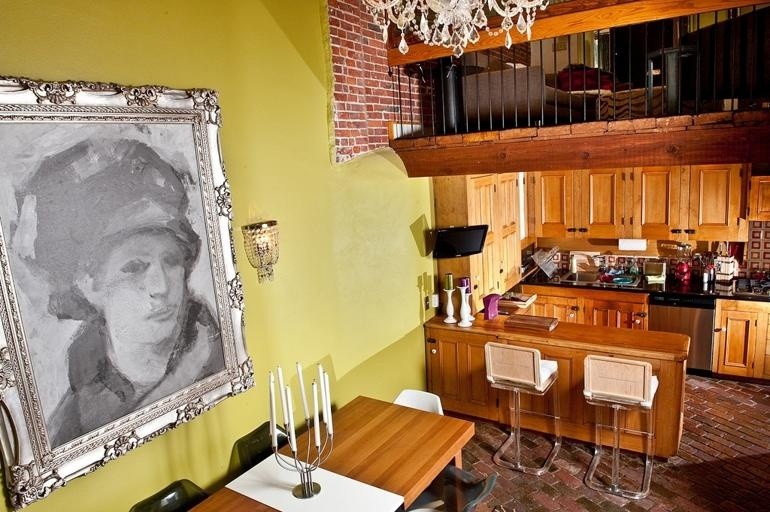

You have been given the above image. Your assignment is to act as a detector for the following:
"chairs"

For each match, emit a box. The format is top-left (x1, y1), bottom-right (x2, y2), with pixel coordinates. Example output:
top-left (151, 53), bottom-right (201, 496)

top-left (441, 46), bottom-right (703, 131)
top-left (582, 355), bottom-right (663, 501)
top-left (395, 388), bottom-right (445, 419)
top-left (238, 422), bottom-right (296, 471)
top-left (484, 339), bottom-right (563, 477)
top-left (128, 477), bottom-right (207, 511)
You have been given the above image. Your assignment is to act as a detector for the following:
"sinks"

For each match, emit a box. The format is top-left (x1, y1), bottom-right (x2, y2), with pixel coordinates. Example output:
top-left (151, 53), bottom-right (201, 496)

top-left (559, 270), bottom-right (600, 283)
top-left (595, 273), bottom-right (642, 287)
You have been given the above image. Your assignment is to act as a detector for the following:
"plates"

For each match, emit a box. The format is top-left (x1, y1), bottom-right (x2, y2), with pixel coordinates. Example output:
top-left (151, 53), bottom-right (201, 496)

top-left (613, 278), bottom-right (633, 283)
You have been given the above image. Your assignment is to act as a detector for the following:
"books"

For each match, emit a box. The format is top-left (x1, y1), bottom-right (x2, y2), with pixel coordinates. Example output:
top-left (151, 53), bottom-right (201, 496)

top-left (504, 313), bottom-right (559, 332)
top-left (499, 291), bottom-right (537, 309)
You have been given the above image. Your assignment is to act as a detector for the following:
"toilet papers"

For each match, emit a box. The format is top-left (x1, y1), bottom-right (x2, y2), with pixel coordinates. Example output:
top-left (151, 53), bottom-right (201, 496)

top-left (570, 256), bottom-right (577, 274)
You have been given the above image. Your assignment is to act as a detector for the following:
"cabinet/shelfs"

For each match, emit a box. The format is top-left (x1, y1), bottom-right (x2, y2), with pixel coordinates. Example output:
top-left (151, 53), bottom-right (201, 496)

top-left (709, 295), bottom-right (770, 386)
top-left (434, 173), bottom-right (532, 317)
top-left (580, 285), bottom-right (650, 333)
top-left (632, 168), bottom-right (754, 243)
top-left (746, 174), bottom-right (770, 222)
top-left (528, 167), bottom-right (631, 238)
top-left (522, 281), bottom-right (580, 324)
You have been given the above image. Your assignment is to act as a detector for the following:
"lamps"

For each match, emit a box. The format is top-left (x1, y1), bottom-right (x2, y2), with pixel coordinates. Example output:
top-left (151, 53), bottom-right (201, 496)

top-left (241, 220), bottom-right (280, 286)
top-left (359, 0), bottom-right (554, 63)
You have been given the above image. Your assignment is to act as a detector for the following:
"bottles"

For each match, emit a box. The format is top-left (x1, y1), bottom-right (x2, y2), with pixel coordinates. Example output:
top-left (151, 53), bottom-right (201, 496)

top-left (710, 267), bottom-right (714, 281)
top-left (702, 269), bottom-right (709, 283)
top-left (522, 259), bottom-right (528, 273)
top-left (703, 282), bottom-right (707, 292)
top-left (709, 283), bottom-right (713, 295)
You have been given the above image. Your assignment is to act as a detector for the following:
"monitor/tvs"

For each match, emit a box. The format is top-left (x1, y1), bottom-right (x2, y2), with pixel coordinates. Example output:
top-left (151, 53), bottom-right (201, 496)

top-left (431, 225), bottom-right (489, 258)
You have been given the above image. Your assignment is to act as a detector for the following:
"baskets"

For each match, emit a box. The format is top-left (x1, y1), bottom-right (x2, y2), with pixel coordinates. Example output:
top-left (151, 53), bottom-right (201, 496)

top-left (717, 256), bottom-right (734, 280)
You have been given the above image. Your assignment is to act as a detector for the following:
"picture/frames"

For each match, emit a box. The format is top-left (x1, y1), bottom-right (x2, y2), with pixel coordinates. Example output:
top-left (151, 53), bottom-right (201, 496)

top-left (0, 73), bottom-right (257, 512)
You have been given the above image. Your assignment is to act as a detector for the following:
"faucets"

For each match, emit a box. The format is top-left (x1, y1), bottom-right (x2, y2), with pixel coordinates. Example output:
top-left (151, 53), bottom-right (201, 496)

top-left (591, 255), bottom-right (608, 272)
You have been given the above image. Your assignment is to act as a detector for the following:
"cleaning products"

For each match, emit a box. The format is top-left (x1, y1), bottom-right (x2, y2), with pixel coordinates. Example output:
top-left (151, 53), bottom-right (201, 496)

top-left (628, 254), bottom-right (640, 275)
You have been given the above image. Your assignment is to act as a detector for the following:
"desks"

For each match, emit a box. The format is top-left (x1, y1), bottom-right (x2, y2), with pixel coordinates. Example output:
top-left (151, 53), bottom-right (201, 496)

top-left (169, 394), bottom-right (478, 512)
top-left (419, 311), bottom-right (694, 463)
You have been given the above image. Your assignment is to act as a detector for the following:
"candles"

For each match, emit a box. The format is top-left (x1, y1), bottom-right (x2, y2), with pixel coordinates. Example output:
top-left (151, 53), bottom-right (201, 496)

top-left (260, 356), bottom-right (337, 455)
top-left (457, 276), bottom-right (466, 288)
top-left (444, 273), bottom-right (454, 290)
top-left (465, 277), bottom-right (472, 294)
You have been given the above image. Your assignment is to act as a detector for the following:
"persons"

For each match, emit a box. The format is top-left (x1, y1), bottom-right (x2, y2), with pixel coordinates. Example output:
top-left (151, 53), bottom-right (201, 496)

top-left (7, 120), bottom-right (226, 453)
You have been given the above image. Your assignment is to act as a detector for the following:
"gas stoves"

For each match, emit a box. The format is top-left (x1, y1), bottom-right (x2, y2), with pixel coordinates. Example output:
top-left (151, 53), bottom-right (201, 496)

top-left (733, 275), bottom-right (770, 297)
